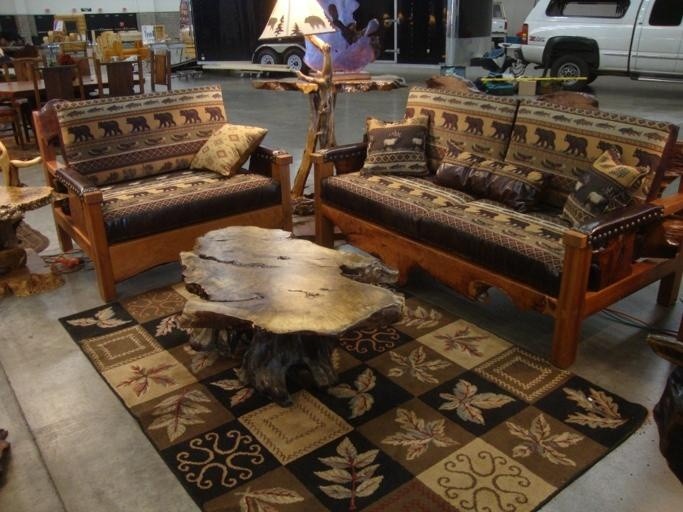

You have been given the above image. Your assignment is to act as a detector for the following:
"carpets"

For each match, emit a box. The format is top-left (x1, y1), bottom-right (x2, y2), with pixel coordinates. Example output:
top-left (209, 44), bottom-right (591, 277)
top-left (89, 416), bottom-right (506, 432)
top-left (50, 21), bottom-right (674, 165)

top-left (57, 280), bottom-right (648, 512)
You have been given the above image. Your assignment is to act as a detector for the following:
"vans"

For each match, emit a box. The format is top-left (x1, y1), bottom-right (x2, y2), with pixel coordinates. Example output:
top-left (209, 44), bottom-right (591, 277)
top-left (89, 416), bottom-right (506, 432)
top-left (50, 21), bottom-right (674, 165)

top-left (515, 0), bottom-right (683, 89)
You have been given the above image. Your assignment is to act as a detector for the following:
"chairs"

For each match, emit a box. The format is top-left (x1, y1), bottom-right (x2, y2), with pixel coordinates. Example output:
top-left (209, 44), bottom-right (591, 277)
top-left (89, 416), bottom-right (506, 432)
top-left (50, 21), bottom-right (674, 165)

top-left (32, 80), bottom-right (295, 306)
top-left (0, 44), bottom-right (171, 150)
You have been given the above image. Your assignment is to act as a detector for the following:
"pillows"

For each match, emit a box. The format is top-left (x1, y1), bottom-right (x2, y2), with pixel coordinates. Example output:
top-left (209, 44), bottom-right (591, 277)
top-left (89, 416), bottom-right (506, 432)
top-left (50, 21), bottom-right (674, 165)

top-left (188, 122), bottom-right (267, 178)
top-left (360, 113), bottom-right (650, 227)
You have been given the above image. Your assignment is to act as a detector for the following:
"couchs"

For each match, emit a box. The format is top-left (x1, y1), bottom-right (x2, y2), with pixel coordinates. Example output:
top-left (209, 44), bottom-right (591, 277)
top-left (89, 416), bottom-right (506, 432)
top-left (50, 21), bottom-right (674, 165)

top-left (309, 78), bottom-right (683, 370)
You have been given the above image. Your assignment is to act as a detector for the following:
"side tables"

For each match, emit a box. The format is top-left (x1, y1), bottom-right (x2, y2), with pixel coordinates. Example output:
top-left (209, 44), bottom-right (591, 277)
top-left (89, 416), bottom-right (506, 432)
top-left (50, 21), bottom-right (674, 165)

top-left (251, 74), bottom-right (407, 214)
top-left (0, 186), bottom-right (67, 303)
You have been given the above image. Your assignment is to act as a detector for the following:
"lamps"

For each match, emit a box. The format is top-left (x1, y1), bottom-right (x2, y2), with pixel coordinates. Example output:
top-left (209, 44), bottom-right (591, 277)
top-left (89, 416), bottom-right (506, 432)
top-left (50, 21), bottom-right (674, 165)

top-left (259, 0), bottom-right (336, 215)
